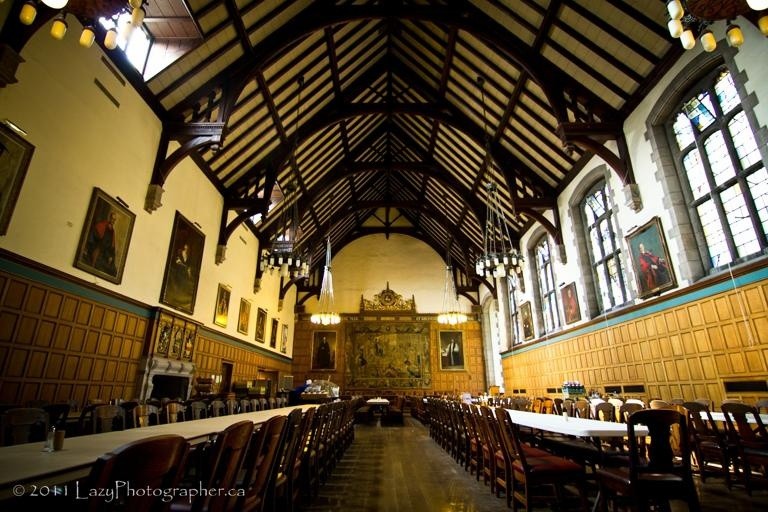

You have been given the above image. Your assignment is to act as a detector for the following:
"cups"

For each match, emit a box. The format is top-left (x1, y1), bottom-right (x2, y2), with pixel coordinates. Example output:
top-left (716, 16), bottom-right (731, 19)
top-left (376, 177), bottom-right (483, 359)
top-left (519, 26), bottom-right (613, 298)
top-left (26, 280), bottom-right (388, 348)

top-left (304, 384), bottom-right (332, 395)
top-left (372, 396), bottom-right (381, 401)
top-left (55, 430), bottom-right (65, 449)
top-left (472, 391), bottom-right (511, 405)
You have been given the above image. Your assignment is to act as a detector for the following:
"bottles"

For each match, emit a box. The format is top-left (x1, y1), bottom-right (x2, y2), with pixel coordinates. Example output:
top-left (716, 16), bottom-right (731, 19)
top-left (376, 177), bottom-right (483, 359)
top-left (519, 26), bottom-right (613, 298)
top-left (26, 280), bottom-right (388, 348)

top-left (44, 423), bottom-right (55, 450)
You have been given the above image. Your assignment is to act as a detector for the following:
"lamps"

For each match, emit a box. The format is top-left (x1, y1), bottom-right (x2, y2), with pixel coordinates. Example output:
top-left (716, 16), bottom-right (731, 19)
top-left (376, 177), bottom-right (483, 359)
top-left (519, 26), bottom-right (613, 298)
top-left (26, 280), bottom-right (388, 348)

top-left (465, 74), bottom-right (524, 281)
top-left (307, 189), bottom-right (342, 326)
top-left (433, 193), bottom-right (466, 327)
top-left (14, 0), bottom-right (147, 50)
top-left (657, 1), bottom-right (767, 55)
top-left (257, 74), bottom-right (312, 279)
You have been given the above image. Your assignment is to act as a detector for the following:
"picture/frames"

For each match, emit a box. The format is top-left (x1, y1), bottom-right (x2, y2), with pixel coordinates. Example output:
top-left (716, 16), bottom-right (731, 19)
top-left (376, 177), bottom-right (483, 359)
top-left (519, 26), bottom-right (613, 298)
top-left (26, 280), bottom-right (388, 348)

top-left (158, 209), bottom-right (207, 318)
top-left (436, 329), bottom-right (466, 372)
top-left (0, 117), bottom-right (35, 241)
top-left (214, 283), bottom-right (289, 356)
top-left (73, 186), bottom-right (136, 287)
top-left (311, 330), bottom-right (336, 370)
top-left (560, 282), bottom-right (582, 326)
top-left (518, 301), bottom-right (536, 341)
top-left (623, 216), bottom-right (674, 299)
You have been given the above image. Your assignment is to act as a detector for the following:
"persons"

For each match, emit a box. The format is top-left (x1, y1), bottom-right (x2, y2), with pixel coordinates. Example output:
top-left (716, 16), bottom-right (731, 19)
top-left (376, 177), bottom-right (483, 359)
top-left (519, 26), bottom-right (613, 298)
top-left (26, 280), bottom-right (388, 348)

top-left (82, 212), bottom-right (118, 278)
top-left (173, 239), bottom-right (193, 289)
top-left (522, 311), bottom-right (532, 338)
top-left (638, 240), bottom-right (670, 289)
top-left (564, 287), bottom-right (578, 323)
top-left (442, 335), bottom-right (461, 366)
top-left (317, 336), bottom-right (331, 366)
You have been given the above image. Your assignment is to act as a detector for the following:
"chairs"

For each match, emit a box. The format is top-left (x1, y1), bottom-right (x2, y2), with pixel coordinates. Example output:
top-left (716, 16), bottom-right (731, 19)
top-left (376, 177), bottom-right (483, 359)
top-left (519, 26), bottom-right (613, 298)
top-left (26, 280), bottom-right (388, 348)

top-left (0, 369), bottom-right (767, 512)
